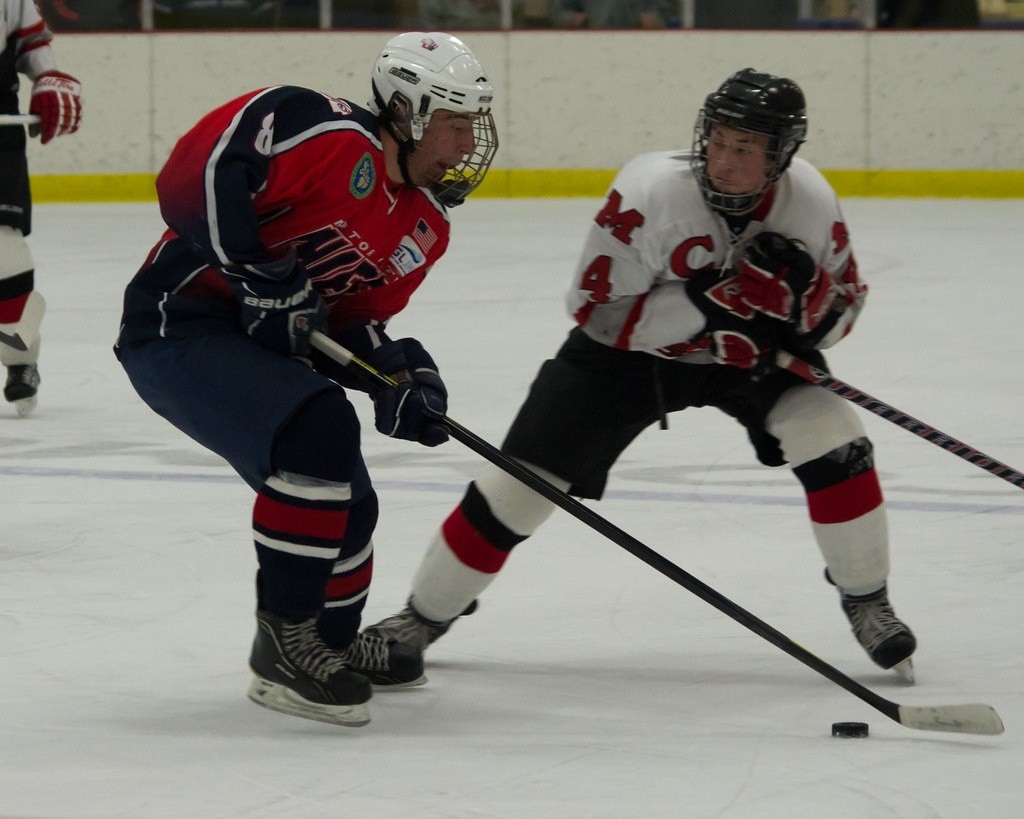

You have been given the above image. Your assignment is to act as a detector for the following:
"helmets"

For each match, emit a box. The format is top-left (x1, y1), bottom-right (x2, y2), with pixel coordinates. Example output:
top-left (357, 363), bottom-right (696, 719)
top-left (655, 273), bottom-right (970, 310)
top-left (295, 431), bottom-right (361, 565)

top-left (689, 67), bottom-right (807, 216)
top-left (366, 31), bottom-right (499, 208)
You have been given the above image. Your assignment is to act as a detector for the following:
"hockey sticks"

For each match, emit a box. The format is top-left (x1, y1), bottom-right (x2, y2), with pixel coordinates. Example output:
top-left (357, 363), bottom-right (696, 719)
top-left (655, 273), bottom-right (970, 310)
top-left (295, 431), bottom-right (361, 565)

top-left (309, 330), bottom-right (1005, 736)
top-left (772, 347), bottom-right (1024, 493)
top-left (0, 113), bottom-right (42, 126)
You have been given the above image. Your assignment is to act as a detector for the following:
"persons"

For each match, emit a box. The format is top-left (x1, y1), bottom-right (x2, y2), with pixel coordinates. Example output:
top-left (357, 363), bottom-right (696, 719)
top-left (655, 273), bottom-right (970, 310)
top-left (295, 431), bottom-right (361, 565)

top-left (112, 29), bottom-right (497, 729)
top-left (363, 65), bottom-right (917, 689)
top-left (35, 0), bottom-right (1023, 30)
top-left (0, 0), bottom-right (82, 417)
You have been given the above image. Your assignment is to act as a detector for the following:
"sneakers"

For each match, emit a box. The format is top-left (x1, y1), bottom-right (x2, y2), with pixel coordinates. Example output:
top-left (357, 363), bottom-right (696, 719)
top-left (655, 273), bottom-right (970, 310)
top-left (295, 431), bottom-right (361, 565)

top-left (245, 568), bottom-right (373, 727)
top-left (824, 565), bottom-right (917, 686)
top-left (363, 593), bottom-right (480, 648)
top-left (319, 591), bottom-right (429, 692)
top-left (3, 363), bottom-right (41, 417)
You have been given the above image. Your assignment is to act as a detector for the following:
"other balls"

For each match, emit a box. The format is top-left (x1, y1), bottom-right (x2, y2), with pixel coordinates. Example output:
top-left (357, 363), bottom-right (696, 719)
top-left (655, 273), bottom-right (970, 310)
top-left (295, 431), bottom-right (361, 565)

top-left (831, 722), bottom-right (870, 739)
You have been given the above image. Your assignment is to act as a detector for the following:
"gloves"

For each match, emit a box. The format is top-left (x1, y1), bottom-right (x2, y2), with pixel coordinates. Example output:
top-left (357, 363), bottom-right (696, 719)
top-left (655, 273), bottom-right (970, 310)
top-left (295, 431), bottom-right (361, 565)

top-left (684, 268), bottom-right (781, 373)
top-left (734, 231), bottom-right (850, 347)
top-left (27, 69), bottom-right (83, 144)
top-left (367, 337), bottom-right (450, 446)
top-left (223, 245), bottom-right (329, 361)
